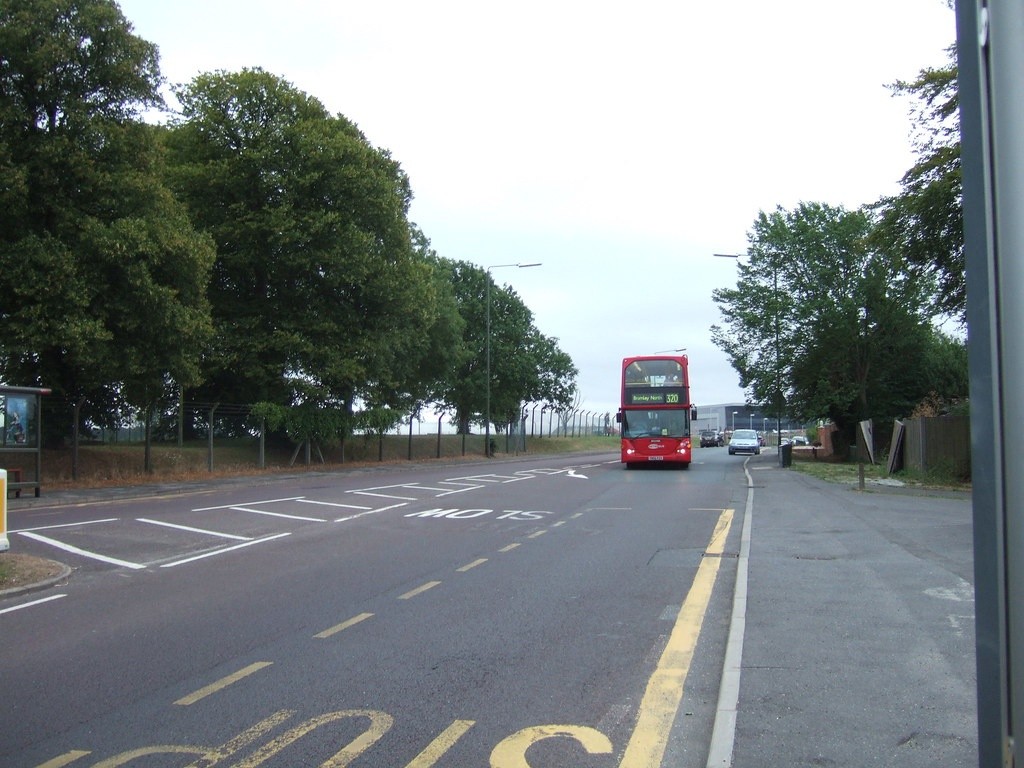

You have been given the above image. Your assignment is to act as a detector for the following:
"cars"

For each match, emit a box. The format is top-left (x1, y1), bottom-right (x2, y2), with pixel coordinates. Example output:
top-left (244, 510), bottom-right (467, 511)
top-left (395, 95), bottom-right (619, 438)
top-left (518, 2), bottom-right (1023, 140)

top-left (791, 436), bottom-right (809, 445)
top-left (757, 435), bottom-right (765, 447)
top-left (727, 429), bottom-right (762, 455)
top-left (780, 438), bottom-right (794, 448)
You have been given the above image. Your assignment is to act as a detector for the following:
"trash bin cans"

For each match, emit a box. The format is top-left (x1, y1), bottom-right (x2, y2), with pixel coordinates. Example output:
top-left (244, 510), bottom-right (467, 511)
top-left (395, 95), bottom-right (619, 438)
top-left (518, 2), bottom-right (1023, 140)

top-left (778, 443), bottom-right (792, 468)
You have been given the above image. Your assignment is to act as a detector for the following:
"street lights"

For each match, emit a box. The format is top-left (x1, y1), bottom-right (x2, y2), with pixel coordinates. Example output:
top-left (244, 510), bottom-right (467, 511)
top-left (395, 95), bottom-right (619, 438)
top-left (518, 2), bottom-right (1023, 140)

top-left (750, 413), bottom-right (754, 429)
top-left (763, 418), bottom-right (767, 432)
top-left (714, 253), bottom-right (782, 448)
top-left (486, 262), bottom-right (542, 456)
top-left (732, 412), bottom-right (737, 431)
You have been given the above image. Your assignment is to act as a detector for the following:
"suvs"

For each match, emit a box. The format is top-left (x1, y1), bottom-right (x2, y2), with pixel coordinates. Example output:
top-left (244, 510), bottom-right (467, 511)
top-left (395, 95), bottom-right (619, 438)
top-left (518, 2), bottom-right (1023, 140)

top-left (700, 431), bottom-right (725, 447)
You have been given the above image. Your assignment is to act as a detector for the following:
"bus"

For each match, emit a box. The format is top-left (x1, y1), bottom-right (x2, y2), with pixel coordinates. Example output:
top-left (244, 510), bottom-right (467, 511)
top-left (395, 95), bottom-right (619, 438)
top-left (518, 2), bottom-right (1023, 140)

top-left (616, 354), bottom-right (699, 468)
